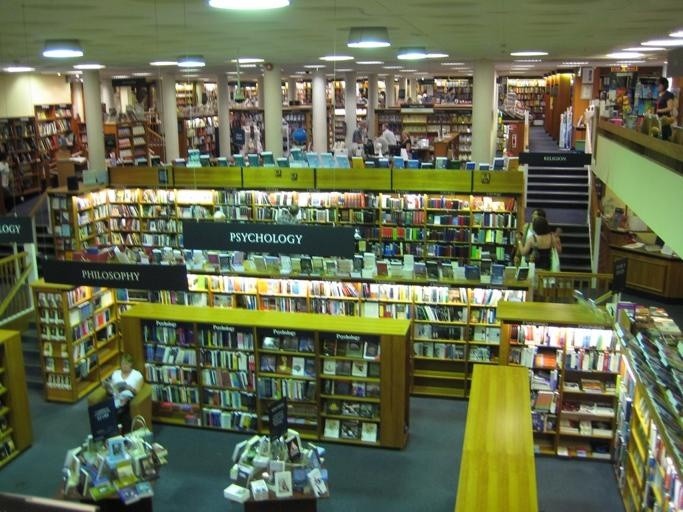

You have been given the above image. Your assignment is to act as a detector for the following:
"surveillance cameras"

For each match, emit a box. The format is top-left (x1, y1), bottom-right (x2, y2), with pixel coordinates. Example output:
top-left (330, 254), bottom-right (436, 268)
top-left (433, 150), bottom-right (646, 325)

top-left (304, 69), bottom-right (309, 73)
top-left (234, 95), bottom-right (245, 103)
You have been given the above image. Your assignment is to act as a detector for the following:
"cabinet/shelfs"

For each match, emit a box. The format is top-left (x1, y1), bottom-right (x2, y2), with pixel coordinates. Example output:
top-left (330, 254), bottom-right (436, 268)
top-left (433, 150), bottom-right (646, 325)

top-left (178, 188), bottom-right (217, 248)
top-left (3, 67), bottom-right (682, 201)
top-left (210, 274), bottom-right (257, 309)
top-left (2, 327), bottom-right (32, 467)
top-left (255, 311), bottom-right (318, 441)
top-left (338, 192), bottom-right (382, 260)
top-left (493, 301), bottom-right (559, 457)
top-left (615, 306), bottom-right (681, 512)
top-left (468, 280), bottom-right (534, 399)
top-left (473, 191), bottom-right (521, 268)
top-left (296, 189), bottom-right (339, 224)
top-left (31, 276), bottom-right (100, 404)
top-left (156, 273), bottom-right (210, 306)
top-left (361, 278), bottom-right (409, 318)
top-left (559, 303), bottom-right (623, 458)
top-left (258, 273), bottom-right (309, 311)
top-left (426, 194), bottom-right (473, 265)
top-left (121, 304), bottom-right (194, 428)
top-left (215, 188), bottom-right (255, 223)
top-left (108, 187), bottom-right (143, 246)
top-left (257, 188), bottom-right (297, 225)
top-left (412, 279), bottom-right (467, 401)
top-left (381, 193), bottom-right (427, 259)
top-left (308, 276), bottom-right (361, 317)
top-left (317, 312), bottom-right (410, 447)
top-left (84, 281), bottom-right (121, 381)
top-left (455, 365), bottom-right (538, 512)
top-left (48, 189), bottom-right (111, 251)
top-left (196, 309), bottom-right (257, 434)
top-left (140, 187), bottom-right (179, 248)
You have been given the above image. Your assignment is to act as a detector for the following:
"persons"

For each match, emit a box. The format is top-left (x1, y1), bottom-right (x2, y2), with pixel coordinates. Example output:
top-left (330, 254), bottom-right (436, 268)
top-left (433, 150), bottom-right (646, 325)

top-left (517, 210), bottom-right (562, 272)
top-left (656, 77), bottom-right (675, 140)
top-left (512, 297), bottom-right (682, 512)
top-left (105, 354), bottom-right (143, 412)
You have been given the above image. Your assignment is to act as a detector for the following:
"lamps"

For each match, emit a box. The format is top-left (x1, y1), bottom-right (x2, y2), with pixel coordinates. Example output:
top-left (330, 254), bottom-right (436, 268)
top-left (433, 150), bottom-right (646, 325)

top-left (42, 39), bottom-right (84, 59)
top-left (348, 26), bottom-right (428, 71)
top-left (175, 54), bottom-right (206, 67)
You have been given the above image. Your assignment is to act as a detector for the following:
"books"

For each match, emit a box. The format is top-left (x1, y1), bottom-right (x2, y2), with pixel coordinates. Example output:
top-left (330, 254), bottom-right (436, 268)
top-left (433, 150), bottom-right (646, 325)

top-left (1, 77), bottom-right (475, 196)
top-left (0, 284), bottom-right (114, 459)
top-left (598, 78), bottom-right (680, 136)
top-left (480, 77), bottom-right (557, 171)
top-left (144, 325), bottom-right (382, 442)
top-left (61, 426), bottom-right (329, 506)
top-left (46, 187), bottom-right (526, 363)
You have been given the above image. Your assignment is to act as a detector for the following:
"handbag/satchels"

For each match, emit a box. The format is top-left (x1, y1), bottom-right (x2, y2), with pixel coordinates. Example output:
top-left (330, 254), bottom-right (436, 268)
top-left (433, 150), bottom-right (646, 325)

top-left (524, 236), bottom-right (540, 264)
